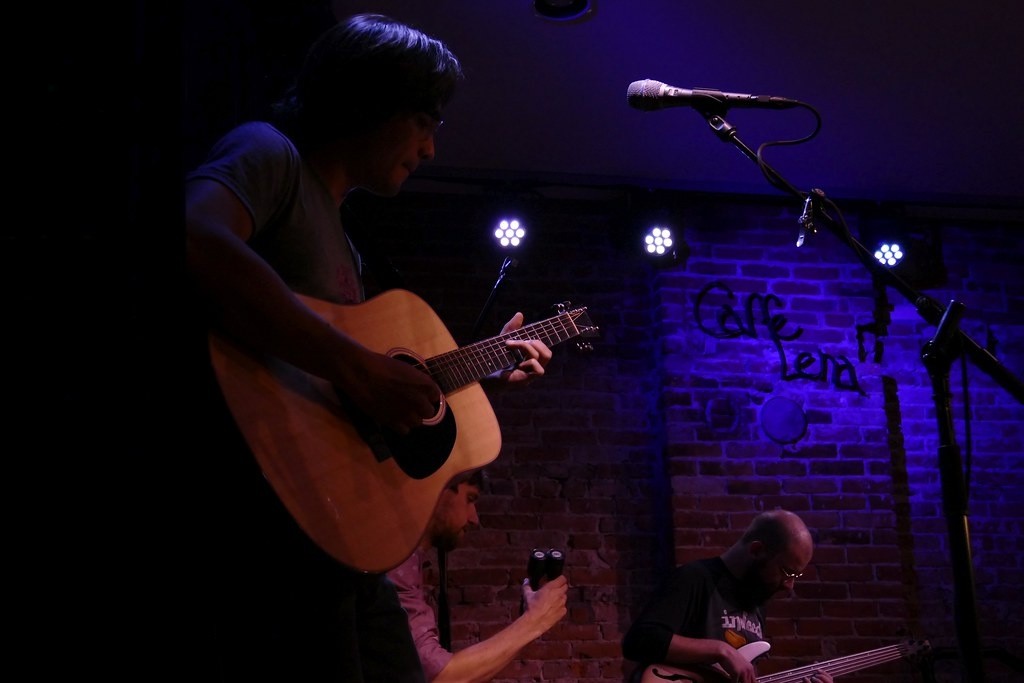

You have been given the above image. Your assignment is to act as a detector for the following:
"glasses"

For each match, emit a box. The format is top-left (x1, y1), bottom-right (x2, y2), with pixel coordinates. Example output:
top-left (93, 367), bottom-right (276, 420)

top-left (766, 554), bottom-right (807, 580)
top-left (423, 107), bottom-right (448, 135)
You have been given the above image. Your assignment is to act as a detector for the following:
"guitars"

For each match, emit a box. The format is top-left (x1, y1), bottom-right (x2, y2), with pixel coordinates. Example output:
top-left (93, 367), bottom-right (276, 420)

top-left (639, 635), bottom-right (931, 683)
top-left (186, 288), bottom-right (604, 576)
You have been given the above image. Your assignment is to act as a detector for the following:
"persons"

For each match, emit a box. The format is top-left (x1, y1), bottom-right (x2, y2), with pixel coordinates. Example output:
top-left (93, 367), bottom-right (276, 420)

top-left (183, 14), bottom-right (553, 682)
top-left (621, 509), bottom-right (834, 683)
top-left (385, 469), bottom-right (570, 682)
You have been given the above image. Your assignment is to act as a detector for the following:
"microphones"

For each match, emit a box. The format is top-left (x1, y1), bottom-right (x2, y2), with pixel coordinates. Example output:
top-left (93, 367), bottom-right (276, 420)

top-left (627, 79), bottom-right (799, 112)
